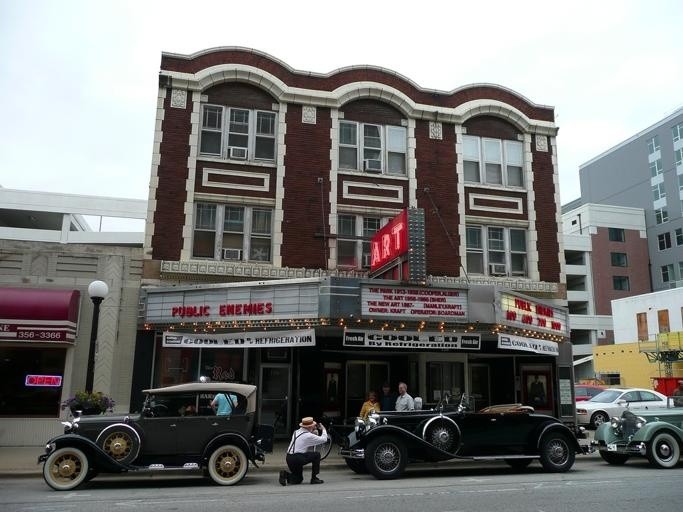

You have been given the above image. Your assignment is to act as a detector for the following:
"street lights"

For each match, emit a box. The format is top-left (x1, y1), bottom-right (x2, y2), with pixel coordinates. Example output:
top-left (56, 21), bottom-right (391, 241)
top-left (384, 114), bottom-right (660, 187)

top-left (84, 280), bottom-right (109, 394)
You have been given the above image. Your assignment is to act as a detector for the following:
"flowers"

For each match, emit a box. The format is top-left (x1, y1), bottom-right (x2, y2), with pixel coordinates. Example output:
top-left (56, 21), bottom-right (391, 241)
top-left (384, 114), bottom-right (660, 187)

top-left (57, 391), bottom-right (116, 418)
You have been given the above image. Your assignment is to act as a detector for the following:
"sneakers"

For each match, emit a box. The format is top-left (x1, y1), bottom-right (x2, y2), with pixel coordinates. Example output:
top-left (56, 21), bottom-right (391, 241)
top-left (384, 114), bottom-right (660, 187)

top-left (278, 469), bottom-right (286, 486)
top-left (310, 477), bottom-right (323, 484)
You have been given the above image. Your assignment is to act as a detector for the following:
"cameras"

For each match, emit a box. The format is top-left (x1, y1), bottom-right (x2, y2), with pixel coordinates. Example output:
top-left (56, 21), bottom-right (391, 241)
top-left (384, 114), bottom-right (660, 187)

top-left (313, 425), bottom-right (320, 430)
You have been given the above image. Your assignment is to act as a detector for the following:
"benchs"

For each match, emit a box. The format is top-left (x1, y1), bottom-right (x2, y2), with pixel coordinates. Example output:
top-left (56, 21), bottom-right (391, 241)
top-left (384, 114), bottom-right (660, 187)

top-left (479, 402), bottom-right (522, 414)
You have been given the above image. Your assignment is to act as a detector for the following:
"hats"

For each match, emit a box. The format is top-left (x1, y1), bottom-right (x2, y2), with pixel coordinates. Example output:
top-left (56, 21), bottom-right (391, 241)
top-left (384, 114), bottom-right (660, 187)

top-left (298, 416), bottom-right (317, 428)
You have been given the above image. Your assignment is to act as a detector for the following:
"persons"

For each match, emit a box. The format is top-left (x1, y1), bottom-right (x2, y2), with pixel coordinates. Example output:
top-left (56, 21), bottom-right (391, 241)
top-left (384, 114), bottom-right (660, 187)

top-left (529, 375), bottom-right (548, 403)
top-left (209, 392), bottom-right (241, 418)
top-left (672, 380), bottom-right (683, 407)
top-left (360, 383), bottom-right (415, 421)
top-left (279, 416), bottom-right (327, 487)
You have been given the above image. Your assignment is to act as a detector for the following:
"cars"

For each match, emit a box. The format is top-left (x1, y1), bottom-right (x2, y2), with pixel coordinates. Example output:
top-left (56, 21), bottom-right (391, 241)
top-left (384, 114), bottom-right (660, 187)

top-left (36, 375), bottom-right (264, 492)
top-left (340, 392), bottom-right (585, 480)
top-left (574, 383), bottom-right (682, 468)
